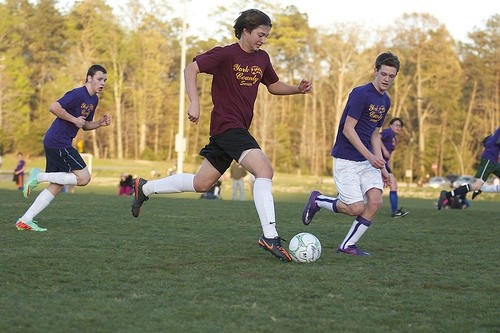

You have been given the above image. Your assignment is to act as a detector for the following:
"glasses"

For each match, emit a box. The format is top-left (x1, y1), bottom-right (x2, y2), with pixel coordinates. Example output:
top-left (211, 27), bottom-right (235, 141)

top-left (392, 123), bottom-right (402, 128)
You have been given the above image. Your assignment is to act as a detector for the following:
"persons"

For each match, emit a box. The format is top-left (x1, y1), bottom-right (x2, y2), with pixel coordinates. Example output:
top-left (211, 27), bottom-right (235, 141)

top-left (303, 52), bottom-right (400, 256)
top-left (131, 8), bottom-right (311, 262)
top-left (433, 192), bottom-right (469, 210)
top-left (200, 180), bottom-right (221, 199)
top-left (119, 174), bottom-right (138, 196)
top-left (230, 159), bottom-right (247, 201)
top-left (15, 152), bottom-right (26, 190)
top-left (16, 65), bottom-right (111, 232)
top-left (379, 117), bottom-right (409, 219)
top-left (438, 126), bottom-right (500, 210)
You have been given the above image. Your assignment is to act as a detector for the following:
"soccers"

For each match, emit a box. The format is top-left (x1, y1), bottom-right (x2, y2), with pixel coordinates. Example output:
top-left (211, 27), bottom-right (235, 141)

top-left (288, 232), bottom-right (322, 263)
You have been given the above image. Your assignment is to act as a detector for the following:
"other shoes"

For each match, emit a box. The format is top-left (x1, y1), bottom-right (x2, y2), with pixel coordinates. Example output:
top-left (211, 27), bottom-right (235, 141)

top-left (472, 189), bottom-right (482, 199)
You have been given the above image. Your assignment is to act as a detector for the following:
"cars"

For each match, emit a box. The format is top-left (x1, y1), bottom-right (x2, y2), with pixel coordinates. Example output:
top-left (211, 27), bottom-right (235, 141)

top-left (424, 175), bottom-right (476, 189)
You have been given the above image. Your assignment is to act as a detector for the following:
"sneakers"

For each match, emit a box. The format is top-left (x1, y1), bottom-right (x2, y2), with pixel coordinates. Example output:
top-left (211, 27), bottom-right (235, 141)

top-left (392, 207), bottom-right (409, 218)
top-left (302, 191), bottom-right (322, 225)
top-left (257, 235), bottom-right (292, 262)
top-left (16, 218), bottom-right (48, 232)
top-left (337, 244), bottom-right (369, 257)
top-left (24, 168), bottom-right (42, 199)
top-left (131, 177), bottom-right (148, 217)
top-left (438, 190), bottom-right (448, 210)
